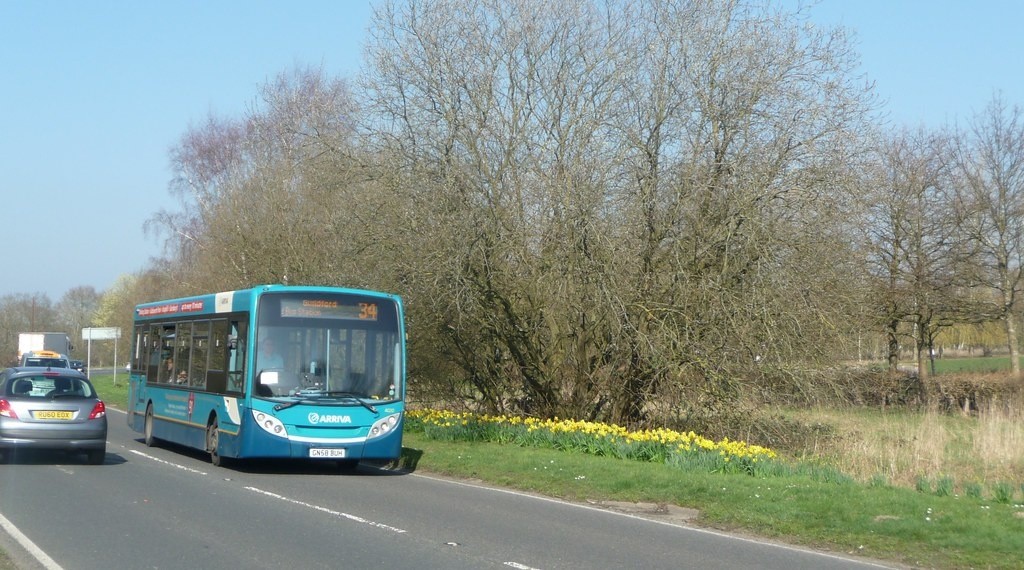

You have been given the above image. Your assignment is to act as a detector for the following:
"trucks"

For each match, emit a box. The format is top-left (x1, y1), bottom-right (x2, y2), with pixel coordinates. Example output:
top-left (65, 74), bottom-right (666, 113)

top-left (18, 332), bottom-right (72, 396)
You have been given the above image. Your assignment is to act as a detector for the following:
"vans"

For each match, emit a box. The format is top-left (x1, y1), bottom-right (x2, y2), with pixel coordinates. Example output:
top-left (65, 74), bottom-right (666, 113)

top-left (21, 350), bottom-right (70, 368)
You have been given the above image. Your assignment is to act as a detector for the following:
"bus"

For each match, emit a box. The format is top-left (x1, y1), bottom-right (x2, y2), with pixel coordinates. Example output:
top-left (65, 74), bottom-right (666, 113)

top-left (127, 284), bottom-right (409, 472)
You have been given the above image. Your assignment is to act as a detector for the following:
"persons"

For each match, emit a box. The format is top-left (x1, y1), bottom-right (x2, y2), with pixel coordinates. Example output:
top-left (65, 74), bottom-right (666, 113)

top-left (152, 335), bottom-right (203, 381)
top-left (256, 337), bottom-right (284, 377)
top-left (46, 379), bottom-right (64, 397)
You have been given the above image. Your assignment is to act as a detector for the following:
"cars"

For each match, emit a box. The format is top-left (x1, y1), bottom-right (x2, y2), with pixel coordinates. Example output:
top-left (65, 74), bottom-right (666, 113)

top-left (0, 366), bottom-right (107, 465)
top-left (71, 361), bottom-right (84, 372)
